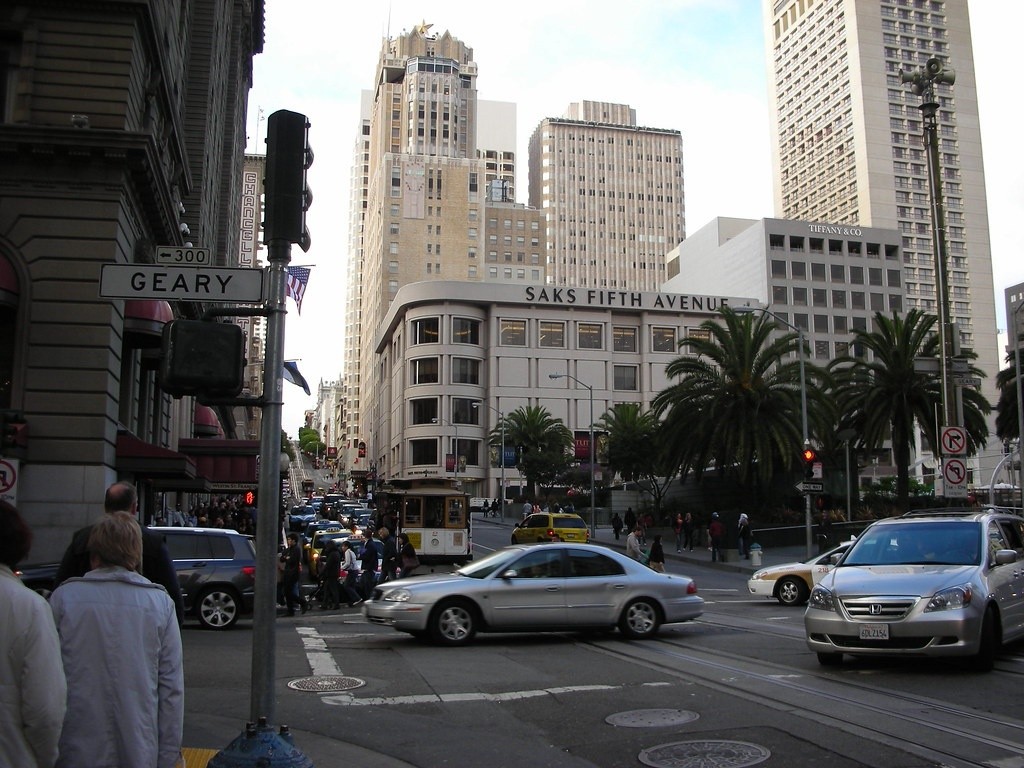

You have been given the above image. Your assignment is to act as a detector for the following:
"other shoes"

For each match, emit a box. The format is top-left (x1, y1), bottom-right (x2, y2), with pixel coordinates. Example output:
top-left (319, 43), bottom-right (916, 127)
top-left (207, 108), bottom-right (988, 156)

top-left (638, 544), bottom-right (642, 546)
top-left (332, 604), bottom-right (340, 610)
top-left (301, 599), bottom-right (309, 615)
top-left (318, 605), bottom-right (327, 609)
top-left (353, 598), bottom-right (363, 606)
top-left (691, 550), bottom-right (694, 552)
top-left (281, 611), bottom-right (295, 617)
top-left (677, 550), bottom-right (681, 553)
top-left (644, 543), bottom-right (647, 546)
top-left (683, 548), bottom-right (686, 551)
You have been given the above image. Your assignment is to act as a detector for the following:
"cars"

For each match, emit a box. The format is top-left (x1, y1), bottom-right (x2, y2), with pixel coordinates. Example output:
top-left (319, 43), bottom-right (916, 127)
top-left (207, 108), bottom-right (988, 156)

top-left (747, 538), bottom-right (900, 606)
top-left (300, 489), bottom-right (373, 520)
top-left (364, 542), bottom-right (703, 649)
top-left (291, 505), bottom-right (385, 584)
top-left (282, 480), bottom-right (291, 509)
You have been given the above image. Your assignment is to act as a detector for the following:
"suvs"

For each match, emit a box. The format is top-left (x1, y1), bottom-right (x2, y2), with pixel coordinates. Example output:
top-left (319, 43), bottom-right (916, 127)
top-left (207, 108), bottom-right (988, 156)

top-left (511, 513), bottom-right (590, 544)
top-left (14, 526), bottom-right (258, 630)
top-left (804, 507), bottom-right (1024, 664)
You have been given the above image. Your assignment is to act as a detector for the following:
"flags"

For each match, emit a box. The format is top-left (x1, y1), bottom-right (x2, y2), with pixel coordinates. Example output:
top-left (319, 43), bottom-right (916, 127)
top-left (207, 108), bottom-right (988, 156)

top-left (287, 266), bottom-right (311, 316)
top-left (283, 362), bottom-right (311, 396)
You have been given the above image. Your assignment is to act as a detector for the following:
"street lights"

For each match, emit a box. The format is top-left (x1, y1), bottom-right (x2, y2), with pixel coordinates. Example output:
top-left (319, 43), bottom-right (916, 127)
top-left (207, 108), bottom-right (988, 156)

top-left (432, 417), bottom-right (458, 480)
top-left (309, 441), bottom-right (319, 469)
top-left (548, 373), bottom-right (596, 539)
top-left (732, 305), bottom-right (814, 560)
top-left (472, 403), bottom-right (509, 524)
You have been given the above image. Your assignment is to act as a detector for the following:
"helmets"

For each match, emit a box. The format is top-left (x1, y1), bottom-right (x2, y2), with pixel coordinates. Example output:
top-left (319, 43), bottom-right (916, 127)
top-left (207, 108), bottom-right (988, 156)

top-left (712, 512), bottom-right (720, 520)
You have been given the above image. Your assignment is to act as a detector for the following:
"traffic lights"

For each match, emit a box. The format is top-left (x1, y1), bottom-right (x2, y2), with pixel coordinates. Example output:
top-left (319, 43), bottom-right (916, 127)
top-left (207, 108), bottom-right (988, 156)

top-left (803, 450), bottom-right (816, 478)
top-left (245, 489), bottom-right (259, 509)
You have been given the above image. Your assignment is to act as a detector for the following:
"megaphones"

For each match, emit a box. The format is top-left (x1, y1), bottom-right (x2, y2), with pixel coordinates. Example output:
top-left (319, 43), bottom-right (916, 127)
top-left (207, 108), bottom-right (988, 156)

top-left (925, 58), bottom-right (942, 78)
top-left (910, 80), bottom-right (929, 96)
top-left (897, 69), bottom-right (920, 86)
top-left (938, 69), bottom-right (956, 87)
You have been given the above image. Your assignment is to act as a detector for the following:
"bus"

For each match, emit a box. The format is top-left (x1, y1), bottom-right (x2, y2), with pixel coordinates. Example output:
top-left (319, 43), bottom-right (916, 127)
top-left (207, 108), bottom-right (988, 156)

top-left (302, 478), bottom-right (315, 492)
top-left (375, 477), bottom-right (474, 566)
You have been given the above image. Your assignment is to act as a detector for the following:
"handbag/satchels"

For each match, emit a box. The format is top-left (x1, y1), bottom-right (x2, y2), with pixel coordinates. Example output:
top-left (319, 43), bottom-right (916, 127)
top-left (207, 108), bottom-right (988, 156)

top-left (289, 557), bottom-right (303, 577)
top-left (335, 568), bottom-right (348, 585)
top-left (339, 550), bottom-right (354, 575)
top-left (400, 543), bottom-right (421, 570)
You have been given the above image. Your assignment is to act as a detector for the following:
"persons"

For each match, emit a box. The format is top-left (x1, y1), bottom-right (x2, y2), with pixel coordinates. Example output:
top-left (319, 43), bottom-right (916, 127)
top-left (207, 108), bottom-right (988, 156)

top-left (48, 481), bottom-right (185, 768)
top-left (809, 502), bottom-right (912, 548)
top-left (523, 500), bottom-right (575, 521)
top-left (482, 498), bottom-right (502, 518)
top-left (0, 499), bottom-right (67, 768)
top-left (144, 496), bottom-right (420, 617)
top-left (610, 506), bottom-right (752, 575)
top-left (318, 464), bottom-right (351, 496)
